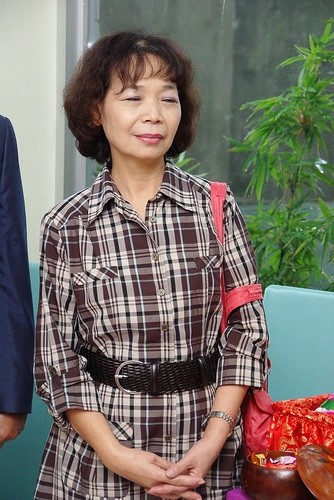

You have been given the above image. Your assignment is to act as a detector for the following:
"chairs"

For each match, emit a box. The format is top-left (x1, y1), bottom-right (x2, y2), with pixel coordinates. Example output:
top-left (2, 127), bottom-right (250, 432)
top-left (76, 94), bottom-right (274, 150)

top-left (263, 285), bottom-right (334, 402)
top-left (0, 262), bottom-right (54, 500)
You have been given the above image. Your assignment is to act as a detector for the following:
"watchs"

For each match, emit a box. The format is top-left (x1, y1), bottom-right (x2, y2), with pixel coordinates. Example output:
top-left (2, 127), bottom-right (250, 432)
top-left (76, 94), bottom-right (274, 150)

top-left (200, 411), bottom-right (235, 439)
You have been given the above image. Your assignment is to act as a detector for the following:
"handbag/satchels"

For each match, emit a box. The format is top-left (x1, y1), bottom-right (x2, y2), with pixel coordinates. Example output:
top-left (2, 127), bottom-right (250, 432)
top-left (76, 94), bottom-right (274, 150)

top-left (209, 181), bottom-right (274, 455)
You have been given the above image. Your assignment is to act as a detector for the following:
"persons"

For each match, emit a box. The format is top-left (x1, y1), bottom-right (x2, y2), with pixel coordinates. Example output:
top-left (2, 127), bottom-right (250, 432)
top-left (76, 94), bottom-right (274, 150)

top-left (32, 32), bottom-right (271, 500)
top-left (0, 115), bottom-right (33, 443)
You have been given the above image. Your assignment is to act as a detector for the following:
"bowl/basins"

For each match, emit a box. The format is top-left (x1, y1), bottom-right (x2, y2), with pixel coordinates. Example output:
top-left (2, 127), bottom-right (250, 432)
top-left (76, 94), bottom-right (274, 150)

top-left (241, 451), bottom-right (305, 500)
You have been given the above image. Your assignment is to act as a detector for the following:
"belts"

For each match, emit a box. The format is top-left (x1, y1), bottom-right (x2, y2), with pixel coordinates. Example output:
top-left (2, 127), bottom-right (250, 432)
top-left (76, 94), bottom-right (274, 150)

top-left (76, 347), bottom-right (221, 396)
